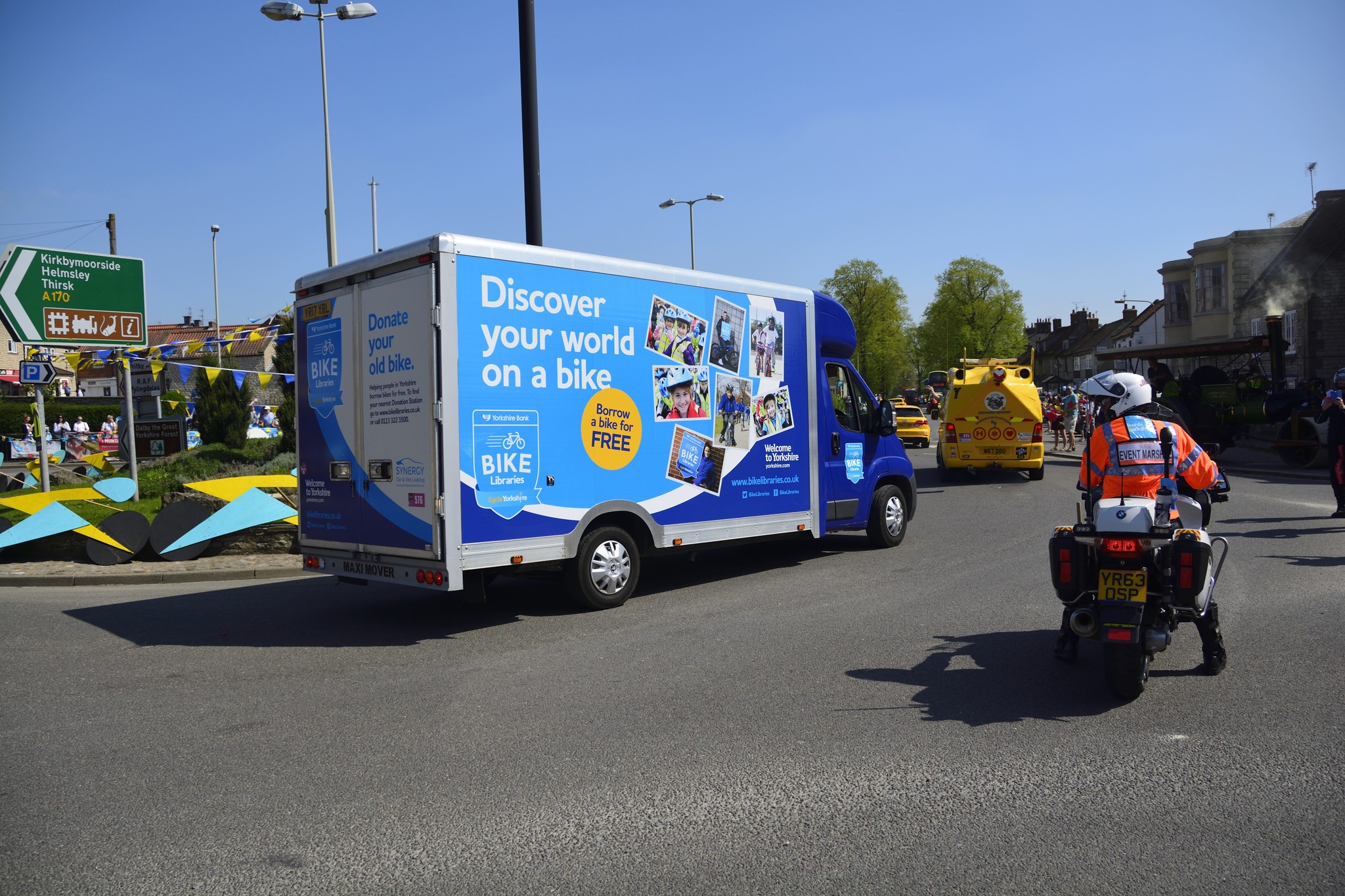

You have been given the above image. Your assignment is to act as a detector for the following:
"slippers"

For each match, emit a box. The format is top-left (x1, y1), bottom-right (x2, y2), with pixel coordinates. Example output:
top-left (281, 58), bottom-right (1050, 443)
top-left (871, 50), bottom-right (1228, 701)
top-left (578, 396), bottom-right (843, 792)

top-left (1044, 429), bottom-right (1054, 433)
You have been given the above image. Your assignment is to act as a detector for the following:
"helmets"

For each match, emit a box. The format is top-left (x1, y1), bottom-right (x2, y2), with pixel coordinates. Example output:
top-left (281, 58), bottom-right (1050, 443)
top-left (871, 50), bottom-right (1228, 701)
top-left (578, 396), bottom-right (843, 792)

top-left (697, 368), bottom-right (708, 382)
top-left (740, 396), bottom-right (743, 399)
top-left (658, 377), bottom-right (669, 398)
top-left (757, 315), bottom-right (776, 327)
top-left (753, 389), bottom-right (787, 409)
top-left (654, 370), bottom-right (659, 377)
top-left (1078, 372), bottom-right (1151, 420)
top-left (665, 366), bottom-right (693, 390)
top-left (1334, 368), bottom-right (1345, 390)
top-left (659, 305), bottom-right (706, 348)
top-left (726, 383), bottom-right (734, 391)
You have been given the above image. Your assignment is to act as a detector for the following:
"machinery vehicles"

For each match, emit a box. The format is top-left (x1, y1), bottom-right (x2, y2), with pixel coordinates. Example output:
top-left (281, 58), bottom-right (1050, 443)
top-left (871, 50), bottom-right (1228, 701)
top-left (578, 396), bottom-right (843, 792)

top-left (1094, 315), bottom-right (1330, 469)
top-left (930, 347), bottom-right (1044, 483)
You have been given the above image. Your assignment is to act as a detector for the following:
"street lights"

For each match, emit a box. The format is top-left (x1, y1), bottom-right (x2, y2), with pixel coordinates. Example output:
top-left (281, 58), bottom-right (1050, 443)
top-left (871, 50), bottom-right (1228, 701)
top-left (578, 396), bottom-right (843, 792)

top-left (658, 193), bottom-right (725, 270)
top-left (260, 0), bottom-right (380, 267)
top-left (1054, 357), bottom-right (1064, 394)
top-left (857, 352), bottom-right (872, 373)
top-left (210, 225), bottom-right (222, 368)
top-left (1114, 300), bottom-right (1159, 363)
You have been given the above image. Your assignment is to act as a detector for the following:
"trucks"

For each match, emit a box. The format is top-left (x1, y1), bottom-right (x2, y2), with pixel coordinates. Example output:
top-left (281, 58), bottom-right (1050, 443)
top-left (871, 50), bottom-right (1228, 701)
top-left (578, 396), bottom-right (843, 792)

top-left (289, 232), bottom-right (918, 610)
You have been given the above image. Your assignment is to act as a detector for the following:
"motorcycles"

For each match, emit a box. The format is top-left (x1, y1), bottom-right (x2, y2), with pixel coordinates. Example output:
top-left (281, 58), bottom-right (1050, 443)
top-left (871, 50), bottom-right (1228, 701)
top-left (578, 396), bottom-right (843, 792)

top-left (1048, 426), bottom-right (1232, 698)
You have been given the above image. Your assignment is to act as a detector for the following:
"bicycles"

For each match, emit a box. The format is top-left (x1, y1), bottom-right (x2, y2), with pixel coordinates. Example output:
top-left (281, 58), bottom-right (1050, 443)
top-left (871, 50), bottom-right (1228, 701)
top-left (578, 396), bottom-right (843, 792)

top-left (710, 337), bottom-right (740, 368)
top-left (738, 411), bottom-right (745, 431)
top-left (718, 410), bottom-right (739, 447)
top-left (758, 341), bottom-right (779, 377)
top-left (778, 401), bottom-right (789, 424)
top-left (754, 414), bottom-right (766, 437)
top-left (751, 339), bottom-right (765, 376)
top-left (746, 414), bottom-right (750, 425)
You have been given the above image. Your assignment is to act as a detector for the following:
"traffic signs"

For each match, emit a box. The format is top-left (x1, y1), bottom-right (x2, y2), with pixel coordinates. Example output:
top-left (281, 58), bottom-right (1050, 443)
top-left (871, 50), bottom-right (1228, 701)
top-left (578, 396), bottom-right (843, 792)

top-left (117, 416), bottom-right (185, 462)
top-left (0, 245), bottom-right (149, 348)
top-left (119, 370), bottom-right (166, 398)
top-left (18, 360), bottom-right (57, 385)
top-left (118, 358), bottom-right (169, 375)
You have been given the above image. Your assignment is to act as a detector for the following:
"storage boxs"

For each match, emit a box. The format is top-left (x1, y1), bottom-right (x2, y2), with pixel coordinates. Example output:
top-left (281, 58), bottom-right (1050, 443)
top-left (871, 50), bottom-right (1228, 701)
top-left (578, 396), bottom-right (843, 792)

top-left (1171, 528), bottom-right (1213, 612)
top-left (1049, 525), bottom-right (1079, 602)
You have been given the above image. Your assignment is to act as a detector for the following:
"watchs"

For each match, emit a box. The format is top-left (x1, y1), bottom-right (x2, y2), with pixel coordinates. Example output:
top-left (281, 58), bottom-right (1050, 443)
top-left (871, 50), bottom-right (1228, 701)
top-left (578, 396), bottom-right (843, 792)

top-left (1340, 405), bottom-right (1345, 409)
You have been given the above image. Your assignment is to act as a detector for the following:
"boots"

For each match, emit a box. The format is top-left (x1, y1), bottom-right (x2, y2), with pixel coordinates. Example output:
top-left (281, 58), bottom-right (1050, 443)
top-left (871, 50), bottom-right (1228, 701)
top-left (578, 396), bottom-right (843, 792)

top-left (1331, 495), bottom-right (1345, 518)
top-left (1194, 604), bottom-right (1226, 674)
top-left (1054, 607), bottom-right (1077, 665)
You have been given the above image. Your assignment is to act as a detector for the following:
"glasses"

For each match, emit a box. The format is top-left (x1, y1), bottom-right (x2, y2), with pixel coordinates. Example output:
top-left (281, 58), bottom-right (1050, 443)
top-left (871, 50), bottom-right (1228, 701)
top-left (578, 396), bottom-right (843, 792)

top-left (78, 419), bottom-right (82, 421)
top-left (666, 319), bottom-right (673, 324)
top-left (58, 417), bottom-right (63, 419)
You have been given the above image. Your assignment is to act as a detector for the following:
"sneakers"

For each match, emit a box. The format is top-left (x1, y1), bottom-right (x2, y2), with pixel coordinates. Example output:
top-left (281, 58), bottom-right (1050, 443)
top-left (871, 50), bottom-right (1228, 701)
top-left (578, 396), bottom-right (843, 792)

top-left (719, 434), bottom-right (725, 443)
top-left (755, 358), bottom-right (775, 372)
top-left (718, 355), bottom-right (729, 365)
top-left (731, 439), bottom-right (737, 445)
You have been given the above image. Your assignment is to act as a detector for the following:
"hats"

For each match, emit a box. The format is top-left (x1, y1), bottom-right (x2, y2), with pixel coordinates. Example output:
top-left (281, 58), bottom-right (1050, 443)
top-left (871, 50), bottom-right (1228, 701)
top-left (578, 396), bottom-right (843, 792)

top-left (264, 406), bottom-right (271, 410)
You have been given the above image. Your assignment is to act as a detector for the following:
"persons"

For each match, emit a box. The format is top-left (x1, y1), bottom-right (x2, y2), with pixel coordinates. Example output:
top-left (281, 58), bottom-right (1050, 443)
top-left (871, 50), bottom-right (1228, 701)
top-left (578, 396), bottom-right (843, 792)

top-left (1147, 357), bottom-right (1174, 394)
top-left (190, 382), bottom-right (202, 402)
top-left (716, 311), bottom-right (731, 366)
top-left (874, 394), bottom-right (882, 402)
top-left (100, 415), bottom-right (118, 433)
top-left (63, 383), bottom-right (71, 397)
top-left (250, 406), bottom-right (259, 428)
top-left (862, 404), bottom-right (866, 414)
top-left (753, 389), bottom-right (791, 437)
top-left (54, 414), bottom-right (71, 439)
top-left (752, 317), bottom-right (780, 377)
top-left (76, 385), bottom-right (86, 397)
top-left (262, 406), bottom-right (277, 427)
top-left (1036, 384), bottom-right (1114, 452)
top-left (1314, 368), bottom-right (1345, 519)
top-left (73, 416), bottom-right (90, 433)
top-left (271, 411), bottom-right (281, 430)
top-left (647, 299), bottom-right (706, 366)
top-left (926, 384), bottom-right (952, 409)
top-left (22, 414), bottom-right (35, 441)
top-left (1052, 369), bottom-right (1227, 676)
top-left (844, 396), bottom-right (852, 416)
top-left (718, 383), bottom-right (750, 447)
top-left (654, 367), bottom-right (710, 420)
top-left (676, 442), bottom-right (716, 490)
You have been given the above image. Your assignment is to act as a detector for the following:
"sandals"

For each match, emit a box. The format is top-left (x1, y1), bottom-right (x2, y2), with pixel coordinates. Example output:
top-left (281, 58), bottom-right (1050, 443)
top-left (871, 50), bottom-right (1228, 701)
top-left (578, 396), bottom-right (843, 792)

top-left (1050, 445), bottom-right (1075, 452)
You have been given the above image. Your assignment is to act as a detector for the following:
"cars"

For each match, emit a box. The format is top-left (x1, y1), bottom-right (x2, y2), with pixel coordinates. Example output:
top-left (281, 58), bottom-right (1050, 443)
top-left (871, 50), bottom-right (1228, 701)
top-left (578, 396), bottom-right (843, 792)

top-left (876, 371), bottom-right (947, 414)
top-left (894, 405), bottom-right (930, 448)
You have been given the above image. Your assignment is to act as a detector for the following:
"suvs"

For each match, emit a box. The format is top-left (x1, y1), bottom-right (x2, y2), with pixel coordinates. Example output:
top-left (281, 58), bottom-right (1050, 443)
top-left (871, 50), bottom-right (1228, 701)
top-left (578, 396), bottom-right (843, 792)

top-left (839, 395), bottom-right (867, 415)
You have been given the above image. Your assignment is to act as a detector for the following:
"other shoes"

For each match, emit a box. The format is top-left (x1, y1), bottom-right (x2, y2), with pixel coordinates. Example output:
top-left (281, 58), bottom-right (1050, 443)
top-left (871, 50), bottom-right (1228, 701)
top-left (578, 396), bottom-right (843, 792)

top-left (1079, 437), bottom-right (1085, 441)
top-left (1067, 440), bottom-right (1069, 443)
